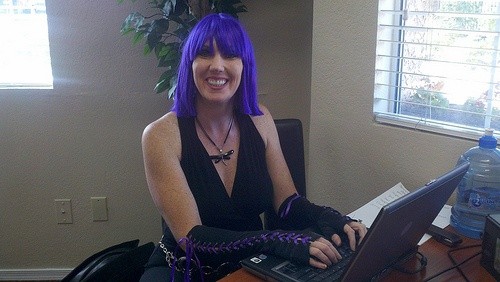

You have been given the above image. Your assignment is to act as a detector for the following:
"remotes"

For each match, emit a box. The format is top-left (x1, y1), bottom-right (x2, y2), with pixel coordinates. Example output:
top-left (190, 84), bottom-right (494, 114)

top-left (427, 224), bottom-right (462, 247)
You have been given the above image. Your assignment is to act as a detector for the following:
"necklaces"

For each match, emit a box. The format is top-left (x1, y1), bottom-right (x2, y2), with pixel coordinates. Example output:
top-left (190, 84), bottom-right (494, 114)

top-left (195, 110), bottom-right (235, 165)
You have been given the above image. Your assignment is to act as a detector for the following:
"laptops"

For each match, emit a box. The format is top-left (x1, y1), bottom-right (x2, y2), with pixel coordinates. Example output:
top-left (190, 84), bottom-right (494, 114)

top-left (240, 161), bottom-right (470, 282)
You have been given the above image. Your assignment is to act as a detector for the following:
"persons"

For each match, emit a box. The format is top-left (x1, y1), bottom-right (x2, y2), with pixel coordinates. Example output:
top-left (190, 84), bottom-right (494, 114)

top-left (140, 13), bottom-right (368, 282)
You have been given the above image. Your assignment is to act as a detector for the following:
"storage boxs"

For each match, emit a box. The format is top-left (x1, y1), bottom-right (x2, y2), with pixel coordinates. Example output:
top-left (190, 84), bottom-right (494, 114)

top-left (479, 213), bottom-right (500, 282)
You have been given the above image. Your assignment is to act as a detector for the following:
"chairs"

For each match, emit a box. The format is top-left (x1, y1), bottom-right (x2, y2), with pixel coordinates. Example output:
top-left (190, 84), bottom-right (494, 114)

top-left (264, 119), bottom-right (307, 231)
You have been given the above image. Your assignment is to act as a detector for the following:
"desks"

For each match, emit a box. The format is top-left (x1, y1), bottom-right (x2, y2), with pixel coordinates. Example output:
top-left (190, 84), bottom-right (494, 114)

top-left (217, 225), bottom-right (500, 282)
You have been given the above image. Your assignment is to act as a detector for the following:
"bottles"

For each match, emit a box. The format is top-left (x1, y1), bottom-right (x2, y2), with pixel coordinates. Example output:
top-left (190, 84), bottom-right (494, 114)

top-left (449, 136), bottom-right (500, 239)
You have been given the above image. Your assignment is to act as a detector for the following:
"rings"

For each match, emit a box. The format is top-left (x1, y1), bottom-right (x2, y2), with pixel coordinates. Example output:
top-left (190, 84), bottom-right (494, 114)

top-left (358, 219), bottom-right (363, 223)
